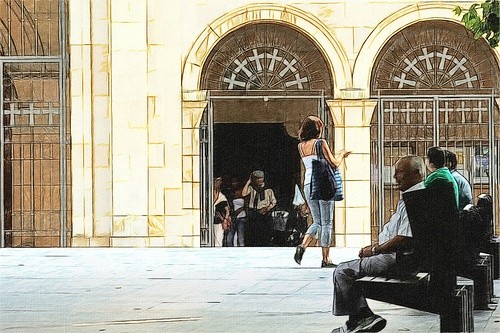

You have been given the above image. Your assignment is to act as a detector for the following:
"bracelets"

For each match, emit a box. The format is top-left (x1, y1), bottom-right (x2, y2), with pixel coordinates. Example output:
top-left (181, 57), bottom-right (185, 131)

top-left (371, 246), bottom-right (375, 255)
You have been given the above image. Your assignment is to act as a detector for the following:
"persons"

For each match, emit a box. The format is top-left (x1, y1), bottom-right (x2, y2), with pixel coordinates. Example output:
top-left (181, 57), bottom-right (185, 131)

top-left (444, 151), bottom-right (472, 210)
top-left (332, 156), bottom-right (426, 333)
top-left (294, 116), bottom-right (352, 267)
top-left (241, 170), bottom-right (277, 247)
top-left (424, 146), bottom-right (459, 226)
top-left (213, 176), bottom-right (246, 247)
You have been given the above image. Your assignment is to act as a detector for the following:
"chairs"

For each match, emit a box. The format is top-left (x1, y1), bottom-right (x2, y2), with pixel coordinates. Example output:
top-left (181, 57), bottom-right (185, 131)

top-left (353, 193), bottom-right (500, 333)
